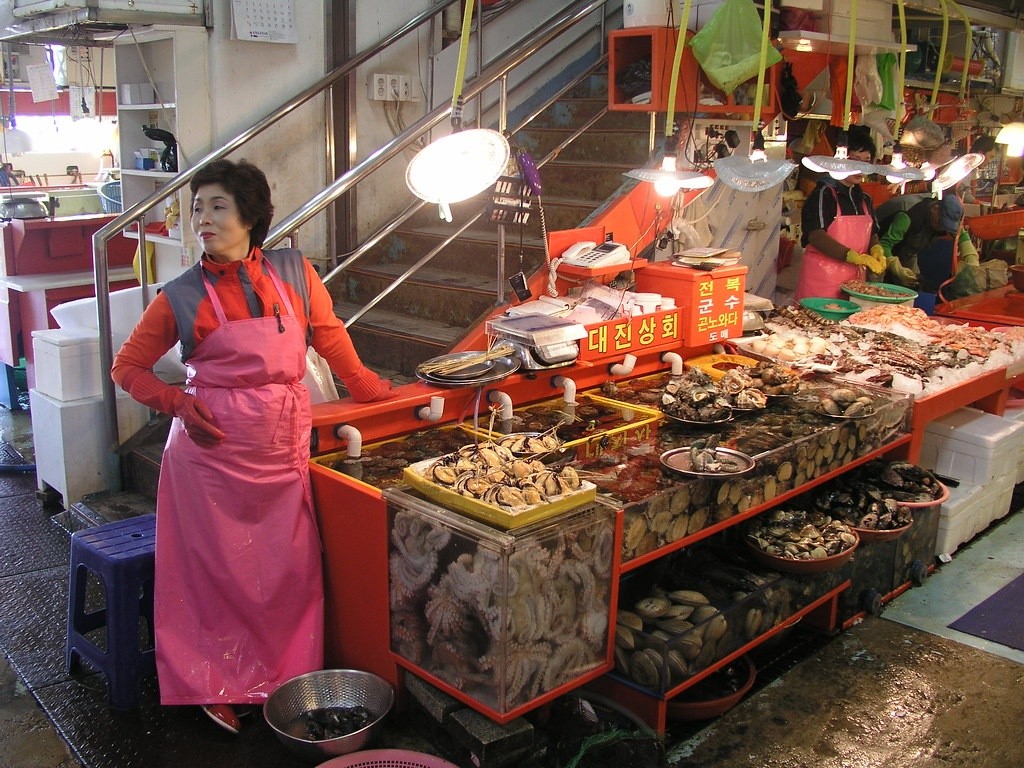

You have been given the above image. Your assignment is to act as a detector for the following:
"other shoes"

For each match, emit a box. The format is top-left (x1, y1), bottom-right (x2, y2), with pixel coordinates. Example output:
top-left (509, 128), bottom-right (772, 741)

top-left (200, 703), bottom-right (240, 733)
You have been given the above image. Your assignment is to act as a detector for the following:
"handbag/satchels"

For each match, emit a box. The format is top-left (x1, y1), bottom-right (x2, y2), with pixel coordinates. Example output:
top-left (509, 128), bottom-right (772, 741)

top-left (688, 0), bottom-right (784, 96)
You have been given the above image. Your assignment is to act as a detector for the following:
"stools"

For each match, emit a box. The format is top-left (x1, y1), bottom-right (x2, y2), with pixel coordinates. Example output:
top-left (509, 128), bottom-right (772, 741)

top-left (65, 513), bottom-right (156, 710)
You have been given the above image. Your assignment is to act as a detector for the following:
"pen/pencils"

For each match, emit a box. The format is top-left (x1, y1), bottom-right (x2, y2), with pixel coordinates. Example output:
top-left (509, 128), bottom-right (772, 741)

top-left (273, 304), bottom-right (285, 333)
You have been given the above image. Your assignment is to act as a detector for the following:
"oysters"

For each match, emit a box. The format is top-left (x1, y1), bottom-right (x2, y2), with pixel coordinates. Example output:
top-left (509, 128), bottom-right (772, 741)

top-left (617, 611), bottom-right (645, 632)
top-left (748, 509), bottom-right (855, 558)
top-left (844, 402), bottom-right (866, 416)
top-left (832, 388), bottom-right (855, 405)
top-left (666, 589), bottom-right (709, 606)
top-left (693, 606), bottom-right (719, 625)
top-left (499, 370), bottom-right (896, 554)
top-left (615, 615), bottom-right (728, 691)
top-left (360, 428), bottom-right (470, 482)
top-left (856, 397), bottom-right (874, 412)
top-left (818, 399), bottom-right (841, 415)
top-left (818, 484), bottom-right (910, 530)
top-left (636, 597), bottom-right (669, 618)
top-left (869, 462), bottom-right (943, 502)
top-left (666, 604), bottom-right (693, 621)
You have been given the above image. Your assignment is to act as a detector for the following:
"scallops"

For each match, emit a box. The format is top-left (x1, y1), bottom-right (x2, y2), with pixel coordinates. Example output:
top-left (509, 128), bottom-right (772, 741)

top-left (733, 569), bottom-right (812, 634)
top-left (428, 440), bottom-right (579, 508)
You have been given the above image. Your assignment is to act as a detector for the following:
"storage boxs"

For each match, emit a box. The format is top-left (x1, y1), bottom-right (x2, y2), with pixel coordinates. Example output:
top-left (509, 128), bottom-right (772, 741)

top-left (309, 282), bottom-right (1024, 744)
top-left (30, 283), bottom-right (178, 399)
top-left (120, 82), bottom-right (155, 104)
top-left (135, 147), bottom-right (163, 171)
top-left (166, 188), bottom-right (180, 239)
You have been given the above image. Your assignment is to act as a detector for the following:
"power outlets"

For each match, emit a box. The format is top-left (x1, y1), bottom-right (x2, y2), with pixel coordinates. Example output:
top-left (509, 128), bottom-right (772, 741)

top-left (367, 74), bottom-right (413, 102)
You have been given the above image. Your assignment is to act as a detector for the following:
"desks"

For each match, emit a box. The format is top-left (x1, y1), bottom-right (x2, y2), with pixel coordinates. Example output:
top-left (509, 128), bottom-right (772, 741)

top-left (0, 214), bottom-right (143, 410)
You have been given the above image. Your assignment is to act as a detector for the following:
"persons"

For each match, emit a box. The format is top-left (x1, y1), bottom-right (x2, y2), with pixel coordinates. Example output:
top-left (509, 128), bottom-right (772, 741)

top-left (110, 157), bottom-right (401, 734)
top-left (0, 156), bottom-right (11, 187)
top-left (875, 194), bottom-right (979, 290)
top-left (794, 133), bottom-right (887, 309)
top-left (948, 136), bottom-right (1000, 205)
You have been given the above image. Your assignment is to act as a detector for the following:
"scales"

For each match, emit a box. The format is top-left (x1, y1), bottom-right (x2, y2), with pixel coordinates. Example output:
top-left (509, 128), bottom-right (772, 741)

top-left (744, 292), bottom-right (775, 331)
top-left (485, 312), bottom-right (588, 370)
top-left (0, 192), bottom-right (50, 220)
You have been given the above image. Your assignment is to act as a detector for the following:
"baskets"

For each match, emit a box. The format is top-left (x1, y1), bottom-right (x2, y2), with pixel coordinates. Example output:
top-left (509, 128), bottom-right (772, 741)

top-left (97, 179), bottom-right (122, 213)
top-left (315, 749), bottom-right (460, 768)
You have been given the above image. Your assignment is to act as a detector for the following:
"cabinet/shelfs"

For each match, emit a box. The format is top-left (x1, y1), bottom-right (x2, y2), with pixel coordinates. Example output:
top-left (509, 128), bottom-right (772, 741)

top-left (112, 23), bottom-right (212, 247)
top-left (310, 367), bottom-right (1021, 742)
top-left (610, 26), bottom-right (777, 112)
top-left (933, 286), bottom-right (1024, 327)
top-left (29, 388), bottom-right (161, 512)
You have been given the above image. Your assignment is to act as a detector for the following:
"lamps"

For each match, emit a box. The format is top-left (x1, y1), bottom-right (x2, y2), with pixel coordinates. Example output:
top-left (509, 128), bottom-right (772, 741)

top-left (621, 1), bottom-right (1024, 195)
top-left (406, 0), bottom-right (511, 204)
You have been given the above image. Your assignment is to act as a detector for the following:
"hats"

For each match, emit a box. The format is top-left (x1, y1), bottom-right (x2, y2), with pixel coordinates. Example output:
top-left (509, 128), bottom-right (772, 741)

top-left (940, 193), bottom-right (963, 232)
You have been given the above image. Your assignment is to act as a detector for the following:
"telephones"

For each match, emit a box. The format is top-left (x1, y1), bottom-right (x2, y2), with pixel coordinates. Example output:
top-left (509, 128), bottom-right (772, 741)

top-left (562, 242), bottom-right (631, 268)
top-left (519, 153), bottom-right (543, 196)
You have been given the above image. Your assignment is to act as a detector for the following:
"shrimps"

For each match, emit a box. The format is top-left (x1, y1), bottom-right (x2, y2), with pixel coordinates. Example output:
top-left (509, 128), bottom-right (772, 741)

top-left (843, 277), bottom-right (914, 299)
top-left (761, 304), bottom-right (1000, 391)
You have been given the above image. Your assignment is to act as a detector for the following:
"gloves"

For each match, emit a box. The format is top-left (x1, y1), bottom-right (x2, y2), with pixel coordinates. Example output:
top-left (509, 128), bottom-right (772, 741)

top-left (846, 249), bottom-right (881, 274)
top-left (906, 255), bottom-right (921, 276)
top-left (128, 372), bottom-right (227, 450)
top-left (886, 255), bottom-right (918, 287)
top-left (342, 362), bottom-right (400, 402)
top-left (870, 244), bottom-right (887, 272)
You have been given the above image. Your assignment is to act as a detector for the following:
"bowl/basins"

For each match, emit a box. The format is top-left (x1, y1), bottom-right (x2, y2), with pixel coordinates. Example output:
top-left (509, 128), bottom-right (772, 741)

top-left (0, 198), bottom-right (49, 220)
top-left (668, 658), bottom-right (756, 725)
top-left (851, 517), bottom-right (913, 543)
top-left (739, 526), bottom-right (860, 573)
top-left (895, 480), bottom-right (949, 510)
top-left (1009, 264), bottom-right (1024, 291)
top-left (263, 669), bottom-right (395, 760)
top-left (799, 297), bottom-right (861, 320)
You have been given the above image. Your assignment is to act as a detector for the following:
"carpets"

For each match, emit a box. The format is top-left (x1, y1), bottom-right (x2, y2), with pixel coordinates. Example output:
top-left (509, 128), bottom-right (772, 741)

top-left (948, 571), bottom-right (1024, 652)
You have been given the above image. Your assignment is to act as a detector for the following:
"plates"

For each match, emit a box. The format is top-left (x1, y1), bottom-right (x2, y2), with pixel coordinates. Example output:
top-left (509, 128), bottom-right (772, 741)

top-left (841, 282), bottom-right (918, 303)
top-left (415, 350), bottom-right (521, 385)
top-left (496, 432), bottom-right (558, 455)
top-left (659, 446), bottom-right (755, 477)
top-left (665, 390), bottom-right (800, 424)
top-left (813, 403), bottom-right (877, 419)
